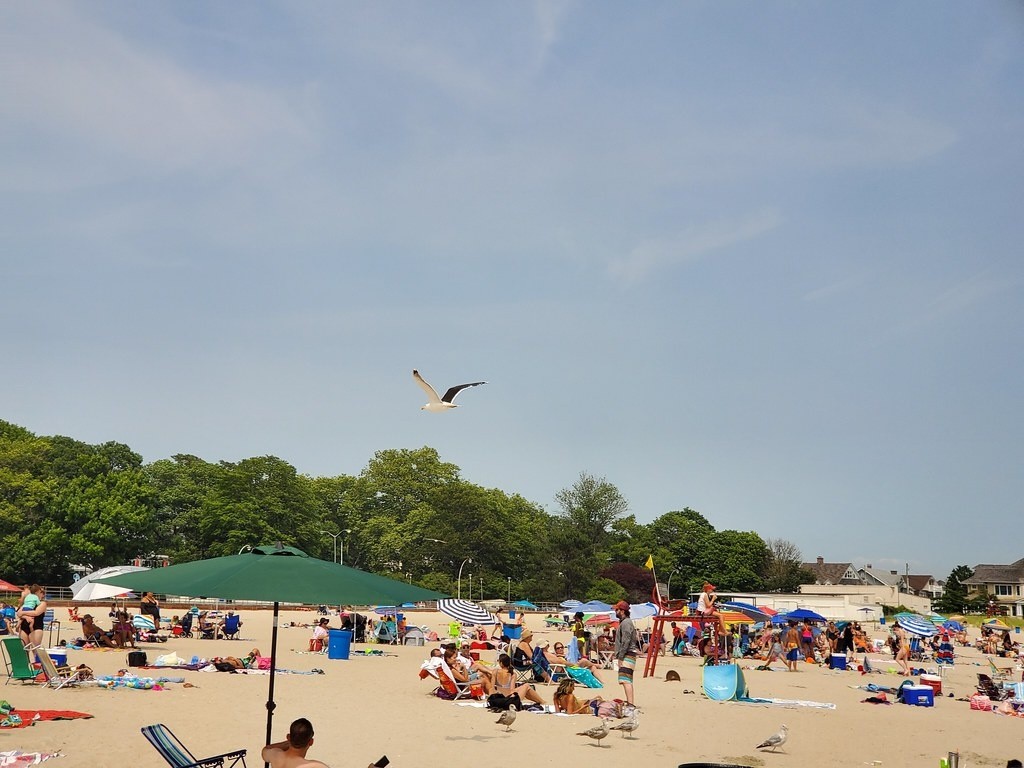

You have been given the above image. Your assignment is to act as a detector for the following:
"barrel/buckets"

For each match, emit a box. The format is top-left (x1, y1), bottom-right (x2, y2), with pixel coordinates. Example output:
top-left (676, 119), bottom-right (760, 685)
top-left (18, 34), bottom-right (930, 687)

top-left (328, 630), bottom-right (352, 660)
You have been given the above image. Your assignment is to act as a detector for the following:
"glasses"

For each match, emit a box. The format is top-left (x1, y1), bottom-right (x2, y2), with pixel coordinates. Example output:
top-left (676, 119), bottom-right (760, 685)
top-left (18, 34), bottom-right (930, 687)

top-left (547, 644), bottom-right (549, 647)
top-left (531, 635), bottom-right (533, 636)
top-left (204, 613), bottom-right (206, 615)
top-left (497, 660), bottom-right (500, 662)
top-left (616, 609), bottom-right (624, 611)
top-left (556, 647), bottom-right (563, 650)
top-left (324, 623), bottom-right (327, 625)
top-left (463, 646), bottom-right (470, 649)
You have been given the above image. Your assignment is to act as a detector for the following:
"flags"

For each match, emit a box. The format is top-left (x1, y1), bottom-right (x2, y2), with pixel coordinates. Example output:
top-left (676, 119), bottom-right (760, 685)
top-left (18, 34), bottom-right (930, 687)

top-left (645, 558), bottom-right (654, 570)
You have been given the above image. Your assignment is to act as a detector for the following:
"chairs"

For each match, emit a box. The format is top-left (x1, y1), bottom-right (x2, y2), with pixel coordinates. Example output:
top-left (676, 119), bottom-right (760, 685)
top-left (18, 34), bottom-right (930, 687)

top-left (309, 614), bottom-right (427, 656)
top-left (987, 656), bottom-right (1016, 682)
top-left (141, 723), bottom-right (248, 768)
top-left (435, 642), bottom-right (616, 702)
top-left (0, 609), bottom-right (242, 691)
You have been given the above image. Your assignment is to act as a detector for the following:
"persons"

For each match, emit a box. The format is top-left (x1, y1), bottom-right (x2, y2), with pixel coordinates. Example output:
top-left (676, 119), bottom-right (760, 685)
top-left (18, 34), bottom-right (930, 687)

top-left (262, 719), bottom-right (380, 768)
top-left (672, 622), bottom-right (872, 672)
top-left (696, 581), bottom-right (732, 636)
top-left (431, 608), bottom-right (671, 715)
top-left (894, 621), bottom-right (910, 677)
top-left (70, 592), bottom-right (262, 683)
top-left (611, 601), bottom-right (637, 710)
top-left (885, 636), bottom-right (942, 659)
top-left (291, 605), bottom-right (407, 651)
top-left (0, 584), bottom-right (48, 662)
top-left (951, 620), bottom-right (1019, 655)
top-left (1006, 760), bottom-right (1022, 768)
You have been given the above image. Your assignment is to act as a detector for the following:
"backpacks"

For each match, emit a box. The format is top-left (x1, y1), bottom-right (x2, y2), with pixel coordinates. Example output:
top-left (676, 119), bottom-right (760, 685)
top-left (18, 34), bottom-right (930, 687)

top-left (898, 681), bottom-right (915, 699)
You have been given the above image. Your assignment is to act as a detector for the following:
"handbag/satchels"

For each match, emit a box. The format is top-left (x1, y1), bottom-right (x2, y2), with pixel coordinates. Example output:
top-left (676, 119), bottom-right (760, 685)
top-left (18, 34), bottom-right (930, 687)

top-left (971, 696), bottom-right (991, 711)
top-left (433, 686), bottom-right (451, 698)
top-left (257, 658), bottom-right (271, 669)
top-left (487, 691), bottom-right (521, 711)
top-left (309, 639), bottom-right (321, 651)
top-left (126, 652), bottom-right (147, 666)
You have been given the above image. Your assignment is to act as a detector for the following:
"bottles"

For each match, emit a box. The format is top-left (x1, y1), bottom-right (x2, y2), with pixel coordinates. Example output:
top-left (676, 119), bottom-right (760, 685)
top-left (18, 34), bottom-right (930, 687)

top-left (949, 751), bottom-right (958, 768)
top-left (938, 665), bottom-right (942, 677)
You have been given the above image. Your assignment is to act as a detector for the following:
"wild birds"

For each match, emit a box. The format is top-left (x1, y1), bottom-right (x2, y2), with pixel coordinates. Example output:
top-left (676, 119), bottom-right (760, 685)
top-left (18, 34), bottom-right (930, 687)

top-left (576, 717), bottom-right (613, 747)
top-left (756, 724), bottom-right (789, 753)
top-left (496, 704), bottom-right (517, 732)
top-left (610, 709), bottom-right (645, 739)
top-left (413, 368), bottom-right (486, 411)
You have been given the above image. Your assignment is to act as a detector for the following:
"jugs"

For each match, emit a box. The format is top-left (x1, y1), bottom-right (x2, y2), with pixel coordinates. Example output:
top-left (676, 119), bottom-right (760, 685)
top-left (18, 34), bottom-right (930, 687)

top-left (940, 757), bottom-right (950, 768)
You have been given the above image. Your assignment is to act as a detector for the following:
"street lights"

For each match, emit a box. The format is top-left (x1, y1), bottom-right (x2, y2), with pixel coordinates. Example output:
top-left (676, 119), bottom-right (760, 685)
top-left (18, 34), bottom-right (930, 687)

top-left (238, 544), bottom-right (251, 555)
top-left (320, 529), bottom-right (352, 563)
top-left (457, 558), bottom-right (473, 599)
top-left (668, 569), bottom-right (680, 608)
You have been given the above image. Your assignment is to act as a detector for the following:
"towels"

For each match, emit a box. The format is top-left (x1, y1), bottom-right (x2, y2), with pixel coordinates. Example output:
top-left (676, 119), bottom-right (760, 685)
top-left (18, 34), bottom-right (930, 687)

top-left (1002, 682), bottom-right (1024, 698)
top-left (0, 750), bottom-right (68, 768)
top-left (450, 702), bottom-right (601, 717)
top-left (564, 636), bottom-right (582, 664)
top-left (0, 700), bottom-right (94, 729)
top-left (136, 659), bottom-right (315, 677)
top-left (420, 647), bottom-right (476, 683)
top-left (739, 697), bottom-right (837, 711)
top-left (860, 693), bottom-right (887, 705)
top-left (564, 666), bottom-right (604, 689)
top-left (531, 646), bottom-right (550, 676)
top-left (862, 683), bottom-right (890, 692)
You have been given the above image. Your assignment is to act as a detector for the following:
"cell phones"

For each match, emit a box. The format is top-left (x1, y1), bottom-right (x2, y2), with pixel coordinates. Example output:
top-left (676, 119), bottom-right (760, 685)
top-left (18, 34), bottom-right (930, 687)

top-left (374, 756), bottom-right (389, 768)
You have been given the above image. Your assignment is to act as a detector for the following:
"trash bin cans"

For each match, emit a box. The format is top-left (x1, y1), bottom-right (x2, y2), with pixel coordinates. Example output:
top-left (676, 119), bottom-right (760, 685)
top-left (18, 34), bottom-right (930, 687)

top-left (328, 629), bottom-right (352, 660)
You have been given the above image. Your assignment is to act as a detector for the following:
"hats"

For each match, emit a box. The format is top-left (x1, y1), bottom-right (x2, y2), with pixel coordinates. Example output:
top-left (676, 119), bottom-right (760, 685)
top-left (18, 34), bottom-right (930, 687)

top-left (611, 601), bottom-right (630, 611)
top-left (84, 614), bottom-right (94, 619)
top-left (706, 585), bottom-right (716, 590)
top-left (536, 639), bottom-right (549, 648)
top-left (461, 641), bottom-right (469, 647)
top-left (520, 629), bottom-right (531, 642)
top-left (228, 610), bottom-right (234, 615)
top-left (319, 618), bottom-right (329, 626)
top-left (200, 611), bottom-right (208, 616)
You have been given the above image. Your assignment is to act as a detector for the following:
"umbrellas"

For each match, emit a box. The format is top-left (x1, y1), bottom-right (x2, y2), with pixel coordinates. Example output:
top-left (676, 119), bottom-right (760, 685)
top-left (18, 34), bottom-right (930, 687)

top-left (672, 602), bottom-right (850, 642)
top-left (88, 542), bottom-right (453, 768)
top-left (0, 579), bottom-right (23, 592)
top-left (69, 566), bottom-right (152, 647)
top-left (893, 612), bottom-right (968, 651)
top-left (510, 600), bottom-right (539, 613)
top-left (368, 602), bottom-right (417, 644)
top-left (561, 598), bottom-right (660, 664)
top-left (437, 599), bottom-right (498, 625)
top-left (983, 617), bottom-right (1012, 634)
